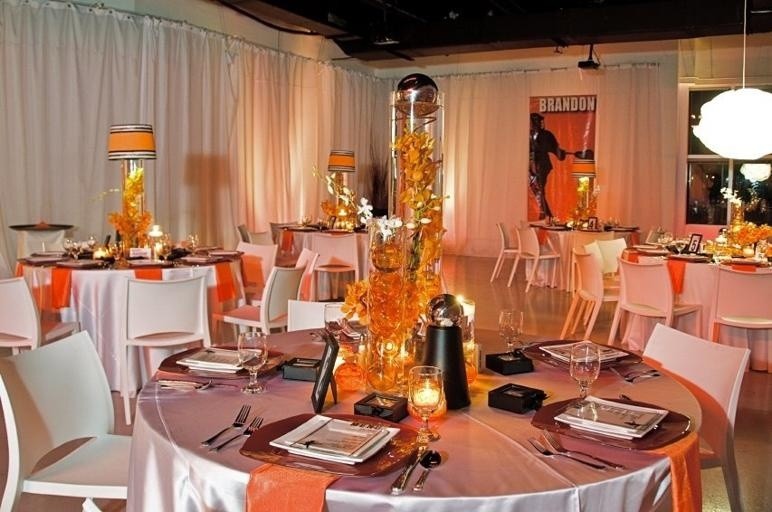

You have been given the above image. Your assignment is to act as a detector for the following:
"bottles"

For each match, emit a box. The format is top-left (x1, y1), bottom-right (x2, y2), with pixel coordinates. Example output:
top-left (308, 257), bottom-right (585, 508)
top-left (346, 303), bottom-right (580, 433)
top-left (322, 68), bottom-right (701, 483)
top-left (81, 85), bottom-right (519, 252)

top-left (425, 324), bottom-right (472, 411)
top-left (334, 355), bottom-right (364, 392)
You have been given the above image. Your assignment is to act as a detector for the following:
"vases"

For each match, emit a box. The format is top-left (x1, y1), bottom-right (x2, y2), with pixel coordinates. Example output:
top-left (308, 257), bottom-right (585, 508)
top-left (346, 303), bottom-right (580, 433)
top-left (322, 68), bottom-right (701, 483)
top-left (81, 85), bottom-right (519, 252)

top-left (392, 73), bottom-right (444, 282)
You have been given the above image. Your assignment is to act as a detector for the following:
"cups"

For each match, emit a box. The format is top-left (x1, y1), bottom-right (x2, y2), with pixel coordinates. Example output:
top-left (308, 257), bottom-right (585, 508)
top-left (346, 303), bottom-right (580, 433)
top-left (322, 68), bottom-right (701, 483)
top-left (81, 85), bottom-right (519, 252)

top-left (574, 183), bottom-right (590, 227)
top-left (728, 200), bottom-right (745, 253)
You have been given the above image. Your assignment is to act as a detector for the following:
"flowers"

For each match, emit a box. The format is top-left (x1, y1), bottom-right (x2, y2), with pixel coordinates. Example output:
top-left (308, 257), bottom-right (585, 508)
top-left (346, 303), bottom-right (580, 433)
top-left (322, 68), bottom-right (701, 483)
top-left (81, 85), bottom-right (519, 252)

top-left (341, 125), bottom-right (448, 369)
top-left (311, 165), bottom-right (373, 229)
top-left (571, 176), bottom-right (600, 218)
top-left (719, 188), bottom-right (772, 249)
top-left (92, 167), bottom-right (152, 260)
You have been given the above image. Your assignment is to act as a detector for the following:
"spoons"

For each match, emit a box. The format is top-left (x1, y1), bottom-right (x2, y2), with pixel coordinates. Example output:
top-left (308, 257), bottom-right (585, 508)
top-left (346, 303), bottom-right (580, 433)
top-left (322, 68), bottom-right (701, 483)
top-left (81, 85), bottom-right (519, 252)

top-left (414, 450), bottom-right (441, 492)
top-left (159, 380), bottom-right (214, 393)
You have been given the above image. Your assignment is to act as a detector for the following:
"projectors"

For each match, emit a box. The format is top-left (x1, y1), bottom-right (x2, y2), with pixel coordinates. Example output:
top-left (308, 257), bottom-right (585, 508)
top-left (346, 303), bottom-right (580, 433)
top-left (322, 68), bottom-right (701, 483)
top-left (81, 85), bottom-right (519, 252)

top-left (578, 61), bottom-right (599, 71)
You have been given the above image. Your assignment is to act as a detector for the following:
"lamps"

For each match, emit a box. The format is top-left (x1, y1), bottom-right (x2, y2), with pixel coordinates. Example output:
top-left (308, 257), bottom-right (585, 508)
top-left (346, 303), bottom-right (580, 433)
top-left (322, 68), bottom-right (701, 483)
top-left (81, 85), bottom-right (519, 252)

top-left (577, 41), bottom-right (600, 71)
top-left (689, 0), bottom-right (772, 161)
top-left (373, 4), bottom-right (400, 46)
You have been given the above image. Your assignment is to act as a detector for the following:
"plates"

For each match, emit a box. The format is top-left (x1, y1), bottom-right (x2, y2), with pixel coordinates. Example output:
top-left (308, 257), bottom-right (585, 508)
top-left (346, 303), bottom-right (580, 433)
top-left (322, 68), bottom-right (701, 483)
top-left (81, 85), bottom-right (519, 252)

top-left (155, 345), bottom-right (294, 377)
top-left (530, 222), bottom-right (638, 231)
top-left (720, 257), bottom-right (762, 265)
top-left (277, 219), bottom-right (416, 234)
top-left (525, 339), bottom-right (697, 451)
top-left (634, 241), bottom-right (665, 255)
top-left (238, 413), bottom-right (420, 479)
top-left (669, 254), bottom-right (708, 260)
top-left (26, 248), bottom-right (235, 269)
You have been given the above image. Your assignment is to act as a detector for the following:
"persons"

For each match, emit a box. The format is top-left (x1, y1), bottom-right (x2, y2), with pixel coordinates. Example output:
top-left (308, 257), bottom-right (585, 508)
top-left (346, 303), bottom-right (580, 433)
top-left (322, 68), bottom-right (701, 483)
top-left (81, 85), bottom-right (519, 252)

top-left (528, 110), bottom-right (568, 220)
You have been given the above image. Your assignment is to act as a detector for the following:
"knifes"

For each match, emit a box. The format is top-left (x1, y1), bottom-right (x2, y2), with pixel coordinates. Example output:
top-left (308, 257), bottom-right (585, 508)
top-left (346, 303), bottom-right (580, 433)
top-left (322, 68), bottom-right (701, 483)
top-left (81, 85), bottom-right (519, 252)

top-left (392, 447), bottom-right (423, 492)
top-left (155, 378), bottom-right (237, 388)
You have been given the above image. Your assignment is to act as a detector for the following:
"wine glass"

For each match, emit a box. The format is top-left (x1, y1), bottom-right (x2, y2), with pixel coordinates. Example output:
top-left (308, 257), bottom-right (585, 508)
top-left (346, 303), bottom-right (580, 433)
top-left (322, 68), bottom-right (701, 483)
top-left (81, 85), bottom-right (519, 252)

top-left (236, 332), bottom-right (270, 395)
top-left (157, 233), bottom-right (174, 260)
top-left (407, 365), bottom-right (442, 442)
top-left (659, 229), bottom-right (772, 254)
top-left (500, 308), bottom-right (523, 356)
top-left (61, 237), bottom-right (83, 262)
top-left (87, 232), bottom-right (98, 256)
top-left (187, 232), bottom-right (198, 257)
top-left (608, 215), bottom-right (620, 228)
top-left (326, 304), bottom-right (349, 344)
top-left (109, 240), bottom-right (129, 265)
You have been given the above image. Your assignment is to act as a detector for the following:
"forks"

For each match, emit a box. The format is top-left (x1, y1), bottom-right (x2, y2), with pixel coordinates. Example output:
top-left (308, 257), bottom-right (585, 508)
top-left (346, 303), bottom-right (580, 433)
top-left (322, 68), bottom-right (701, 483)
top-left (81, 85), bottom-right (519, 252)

top-left (209, 415), bottom-right (265, 453)
top-left (201, 404), bottom-right (250, 446)
top-left (529, 429), bottom-right (627, 474)
